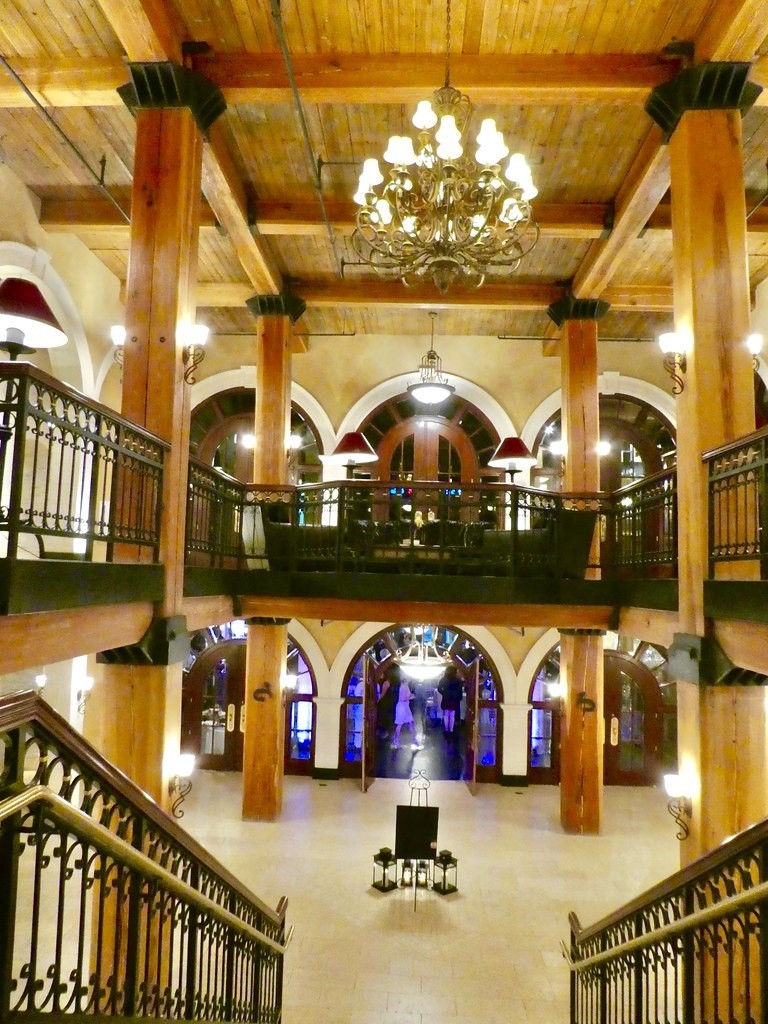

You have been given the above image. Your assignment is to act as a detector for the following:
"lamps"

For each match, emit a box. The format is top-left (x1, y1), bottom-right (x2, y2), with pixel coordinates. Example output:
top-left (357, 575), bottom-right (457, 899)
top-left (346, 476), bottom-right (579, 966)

top-left (407, 309), bottom-right (457, 406)
top-left (281, 675), bottom-right (300, 708)
top-left (108, 322), bottom-right (129, 384)
top-left (664, 774), bottom-right (692, 840)
top-left (488, 437), bottom-right (538, 531)
top-left (36, 676), bottom-right (48, 697)
top-left (183, 324), bottom-right (211, 384)
top-left (0, 310), bottom-right (69, 505)
top-left (348, 0), bottom-right (542, 293)
top-left (748, 334), bottom-right (763, 372)
top-left (392, 625), bottom-right (453, 682)
top-left (75, 675), bottom-right (94, 712)
top-left (331, 432), bottom-right (379, 527)
top-left (170, 754), bottom-right (194, 820)
top-left (659, 331), bottom-right (690, 395)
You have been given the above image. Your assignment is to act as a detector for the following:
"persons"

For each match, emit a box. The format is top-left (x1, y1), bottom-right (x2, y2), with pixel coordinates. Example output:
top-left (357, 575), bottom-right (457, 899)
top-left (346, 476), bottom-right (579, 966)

top-left (354, 675), bottom-right (422, 752)
top-left (436, 666), bottom-right (463, 736)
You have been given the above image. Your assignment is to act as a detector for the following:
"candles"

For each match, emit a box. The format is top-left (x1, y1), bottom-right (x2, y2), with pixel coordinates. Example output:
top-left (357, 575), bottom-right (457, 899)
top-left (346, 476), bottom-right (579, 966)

top-left (419, 873), bottom-right (425, 885)
top-left (383, 869), bottom-right (389, 887)
top-left (404, 871), bottom-right (411, 884)
top-left (441, 872), bottom-right (449, 890)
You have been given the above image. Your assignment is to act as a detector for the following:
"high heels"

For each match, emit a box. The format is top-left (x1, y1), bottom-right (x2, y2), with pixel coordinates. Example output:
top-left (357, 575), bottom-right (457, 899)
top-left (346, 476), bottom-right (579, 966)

top-left (393, 740), bottom-right (401, 748)
top-left (412, 742), bottom-right (419, 746)
